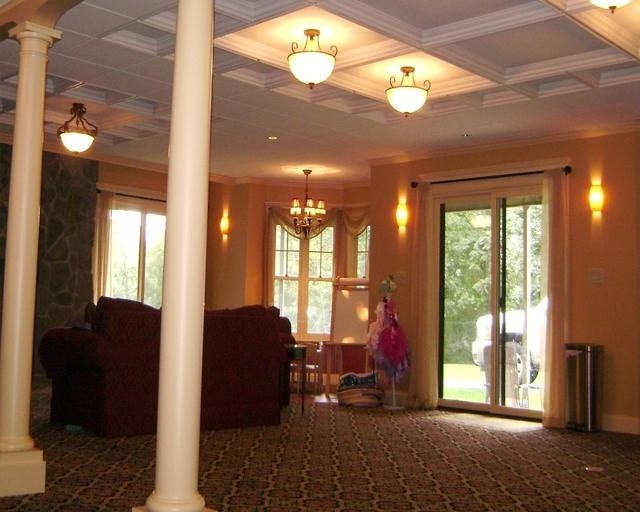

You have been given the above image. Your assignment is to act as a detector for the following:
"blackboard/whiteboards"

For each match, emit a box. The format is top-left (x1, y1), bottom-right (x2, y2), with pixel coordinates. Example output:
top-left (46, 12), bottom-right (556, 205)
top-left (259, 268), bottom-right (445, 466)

top-left (329, 285), bottom-right (370, 347)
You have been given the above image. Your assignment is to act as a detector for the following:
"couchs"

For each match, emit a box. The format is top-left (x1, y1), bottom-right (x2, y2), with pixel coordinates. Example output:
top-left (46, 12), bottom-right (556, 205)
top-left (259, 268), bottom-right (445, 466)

top-left (37, 289), bottom-right (292, 437)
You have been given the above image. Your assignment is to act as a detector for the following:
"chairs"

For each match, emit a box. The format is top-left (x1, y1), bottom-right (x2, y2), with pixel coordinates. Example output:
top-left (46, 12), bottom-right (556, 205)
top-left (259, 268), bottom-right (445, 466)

top-left (290, 340), bottom-right (325, 398)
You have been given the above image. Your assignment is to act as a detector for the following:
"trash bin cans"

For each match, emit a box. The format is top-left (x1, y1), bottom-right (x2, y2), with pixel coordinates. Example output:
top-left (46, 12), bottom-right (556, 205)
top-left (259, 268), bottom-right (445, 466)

top-left (559, 341), bottom-right (606, 435)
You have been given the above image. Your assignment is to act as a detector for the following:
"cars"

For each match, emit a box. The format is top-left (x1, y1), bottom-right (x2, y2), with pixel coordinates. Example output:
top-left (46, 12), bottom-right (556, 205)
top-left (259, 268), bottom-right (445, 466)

top-left (471, 294), bottom-right (548, 385)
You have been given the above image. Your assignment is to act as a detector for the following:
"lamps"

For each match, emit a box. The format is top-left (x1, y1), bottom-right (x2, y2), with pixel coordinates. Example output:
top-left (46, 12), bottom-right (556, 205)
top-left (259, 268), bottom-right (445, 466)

top-left (385, 62), bottom-right (431, 117)
top-left (287, 28), bottom-right (340, 90)
top-left (286, 166), bottom-right (329, 238)
top-left (57, 99), bottom-right (100, 155)
